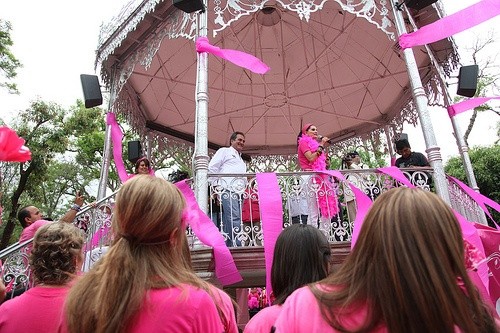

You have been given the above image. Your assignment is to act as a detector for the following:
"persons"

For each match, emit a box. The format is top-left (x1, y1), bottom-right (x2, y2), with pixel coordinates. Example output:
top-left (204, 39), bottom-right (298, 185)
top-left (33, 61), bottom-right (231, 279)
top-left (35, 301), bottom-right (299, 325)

top-left (208, 131), bottom-right (250, 247)
top-left (241, 224), bottom-right (339, 333)
top-left (274, 185), bottom-right (500, 333)
top-left (18, 190), bottom-right (85, 287)
top-left (135, 157), bottom-right (155, 176)
top-left (0, 221), bottom-right (89, 333)
top-left (58, 174), bottom-right (240, 333)
top-left (286, 123), bottom-right (434, 241)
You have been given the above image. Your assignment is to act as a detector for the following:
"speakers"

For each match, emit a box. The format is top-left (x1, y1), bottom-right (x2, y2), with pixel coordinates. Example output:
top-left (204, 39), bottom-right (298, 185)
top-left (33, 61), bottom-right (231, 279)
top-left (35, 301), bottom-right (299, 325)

top-left (457, 65), bottom-right (478, 97)
top-left (80, 74), bottom-right (102, 108)
top-left (128, 140), bottom-right (142, 164)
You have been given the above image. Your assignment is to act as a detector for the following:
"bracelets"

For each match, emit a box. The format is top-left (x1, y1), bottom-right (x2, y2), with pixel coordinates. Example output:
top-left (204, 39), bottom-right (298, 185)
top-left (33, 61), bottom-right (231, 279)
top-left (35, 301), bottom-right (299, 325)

top-left (71, 204), bottom-right (82, 211)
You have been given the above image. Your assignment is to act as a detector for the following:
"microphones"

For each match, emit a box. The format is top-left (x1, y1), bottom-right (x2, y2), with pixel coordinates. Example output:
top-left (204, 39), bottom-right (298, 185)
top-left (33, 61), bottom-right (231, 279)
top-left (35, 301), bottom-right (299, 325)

top-left (318, 135), bottom-right (332, 144)
